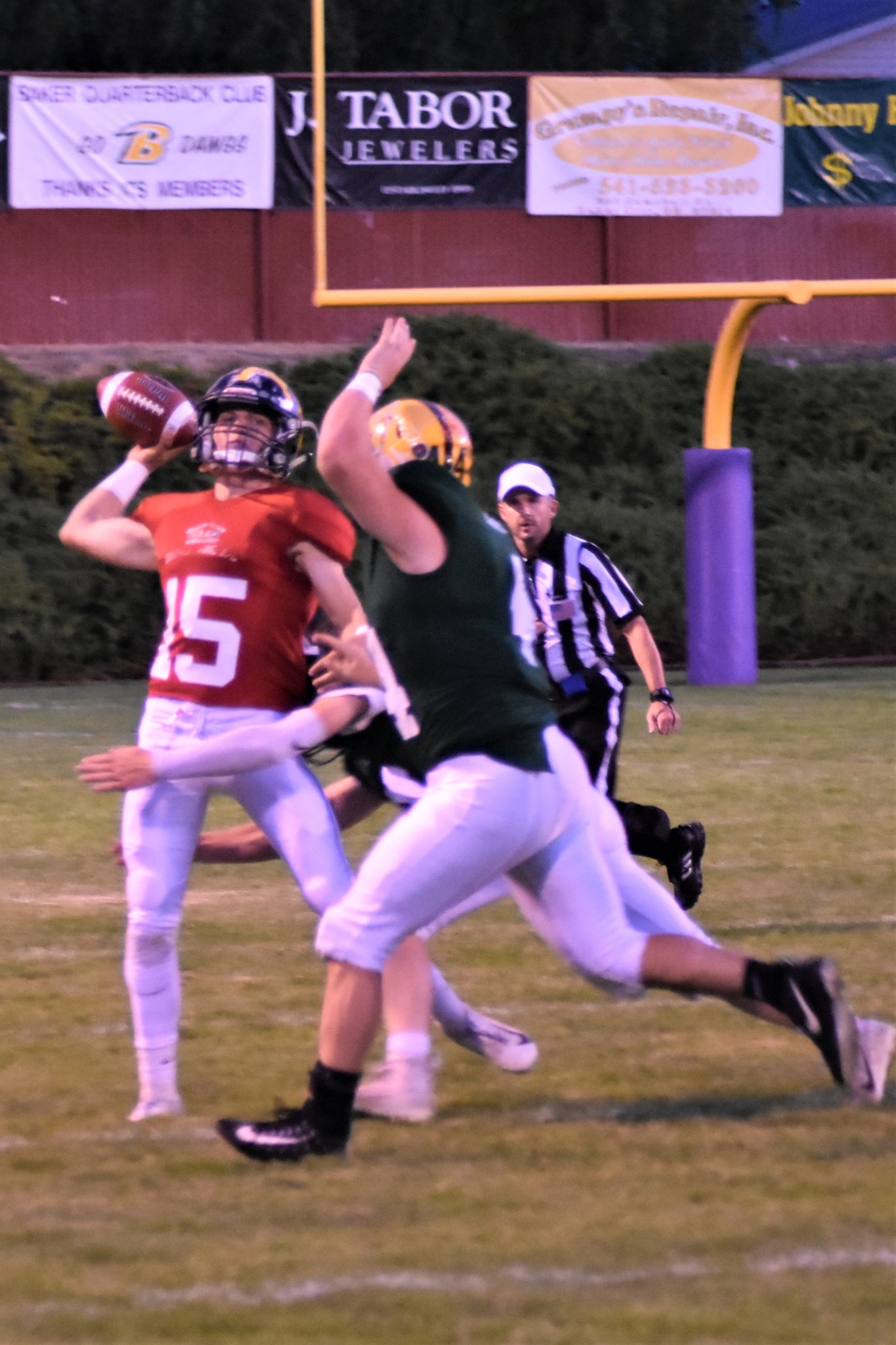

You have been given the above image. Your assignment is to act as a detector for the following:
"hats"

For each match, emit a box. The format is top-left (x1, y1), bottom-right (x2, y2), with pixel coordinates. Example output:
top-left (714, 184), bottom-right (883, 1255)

top-left (497, 463), bottom-right (555, 501)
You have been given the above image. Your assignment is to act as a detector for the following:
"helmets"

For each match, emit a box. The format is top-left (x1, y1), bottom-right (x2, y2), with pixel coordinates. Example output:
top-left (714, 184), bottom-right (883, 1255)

top-left (192, 367), bottom-right (302, 479)
top-left (369, 398), bottom-right (472, 487)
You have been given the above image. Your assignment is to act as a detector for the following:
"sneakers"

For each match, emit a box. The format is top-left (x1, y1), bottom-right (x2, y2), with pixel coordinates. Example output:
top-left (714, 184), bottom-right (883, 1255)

top-left (665, 821), bottom-right (707, 911)
top-left (356, 1051), bottom-right (438, 1123)
top-left (772, 957), bottom-right (858, 1084)
top-left (214, 1097), bottom-right (350, 1163)
top-left (855, 1016), bottom-right (896, 1106)
top-left (441, 1002), bottom-right (537, 1074)
top-left (126, 1098), bottom-right (185, 1122)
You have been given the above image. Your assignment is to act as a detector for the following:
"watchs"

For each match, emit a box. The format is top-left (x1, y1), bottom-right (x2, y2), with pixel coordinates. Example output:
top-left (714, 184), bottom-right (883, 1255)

top-left (650, 687), bottom-right (674, 703)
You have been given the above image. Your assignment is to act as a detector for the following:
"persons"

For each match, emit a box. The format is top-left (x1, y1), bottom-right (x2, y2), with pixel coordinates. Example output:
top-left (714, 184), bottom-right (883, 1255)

top-left (212, 313), bottom-right (859, 1163)
top-left (72, 608), bottom-right (896, 1126)
top-left (59, 366), bottom-right (540, 1126)
top-left (497, 462), bottom-right (705, 911)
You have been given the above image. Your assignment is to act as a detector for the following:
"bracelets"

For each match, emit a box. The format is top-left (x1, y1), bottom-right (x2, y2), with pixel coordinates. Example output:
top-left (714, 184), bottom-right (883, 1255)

top-left (342, 372), bottom-right (382, 406)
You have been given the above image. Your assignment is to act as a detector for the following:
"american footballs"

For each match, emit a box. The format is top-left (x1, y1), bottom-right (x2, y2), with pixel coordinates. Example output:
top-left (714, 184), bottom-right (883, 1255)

top-left (96, 370), bottom-right (199, 449)
